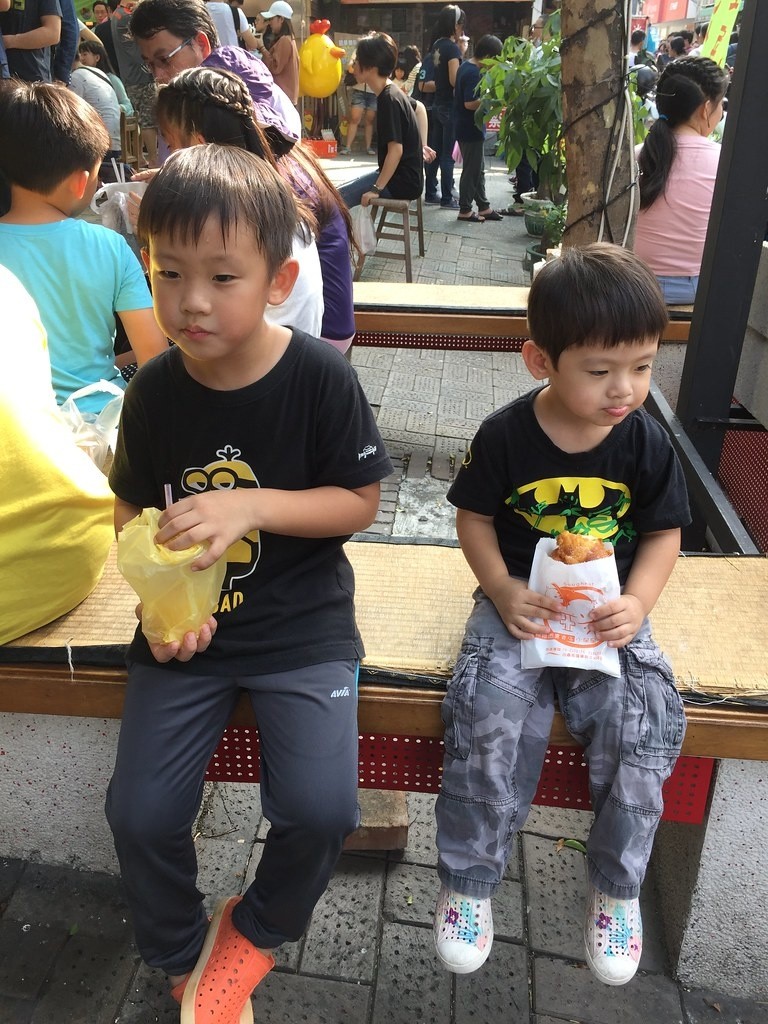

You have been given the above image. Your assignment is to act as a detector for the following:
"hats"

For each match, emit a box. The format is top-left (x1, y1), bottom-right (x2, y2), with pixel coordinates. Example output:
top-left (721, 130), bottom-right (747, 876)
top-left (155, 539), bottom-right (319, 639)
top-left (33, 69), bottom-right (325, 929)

top-left (459, 31), bottom-right (470, 40)
top-left (260, 0), bottom-right (294, 19)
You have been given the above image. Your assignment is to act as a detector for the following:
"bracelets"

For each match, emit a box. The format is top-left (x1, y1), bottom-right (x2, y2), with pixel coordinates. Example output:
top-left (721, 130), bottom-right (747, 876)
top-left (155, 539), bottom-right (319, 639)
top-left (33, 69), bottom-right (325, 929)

top-left (370, 183), bottom-right (383, 194)
top-left (258, 45), bottom-right (265, 51)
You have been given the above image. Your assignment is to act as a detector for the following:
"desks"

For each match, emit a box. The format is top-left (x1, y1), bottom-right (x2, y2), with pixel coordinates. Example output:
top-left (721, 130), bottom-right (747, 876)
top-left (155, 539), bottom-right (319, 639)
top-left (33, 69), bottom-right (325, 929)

top-left (321, 160), bottom-right (380, 208)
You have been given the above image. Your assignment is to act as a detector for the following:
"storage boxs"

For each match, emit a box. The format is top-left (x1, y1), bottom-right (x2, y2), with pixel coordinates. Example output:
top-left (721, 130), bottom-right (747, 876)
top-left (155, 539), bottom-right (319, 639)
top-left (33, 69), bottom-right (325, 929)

top-left (302, 140), bottom-right (337, 158)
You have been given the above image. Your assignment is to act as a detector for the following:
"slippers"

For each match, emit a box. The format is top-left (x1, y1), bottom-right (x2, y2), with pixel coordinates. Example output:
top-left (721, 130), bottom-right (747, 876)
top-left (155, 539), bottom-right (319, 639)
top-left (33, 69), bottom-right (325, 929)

top-left (479, 210), bottom-right (503, 219)
top-left (495, 207), bottom-right (524, 216)
top-left (457, 212), bottom-right (485, 221)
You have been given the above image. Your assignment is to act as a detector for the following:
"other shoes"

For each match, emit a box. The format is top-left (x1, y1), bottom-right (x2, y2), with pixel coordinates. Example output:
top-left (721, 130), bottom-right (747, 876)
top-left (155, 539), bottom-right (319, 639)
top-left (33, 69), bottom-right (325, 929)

top-left (509, 177), bottom-right (518, 183)
top-left (340, 146), bottom-right (351, 155)
top-left (367, 147), bottom-right (375, 155)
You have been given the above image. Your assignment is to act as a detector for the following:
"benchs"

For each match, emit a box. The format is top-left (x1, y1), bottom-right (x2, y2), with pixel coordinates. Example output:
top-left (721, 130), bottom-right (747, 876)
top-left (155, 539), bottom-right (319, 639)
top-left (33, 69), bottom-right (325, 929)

top-left (350, 281), bottom-right (698, 350)
top-left (1, 539), bottom-right (767, 995)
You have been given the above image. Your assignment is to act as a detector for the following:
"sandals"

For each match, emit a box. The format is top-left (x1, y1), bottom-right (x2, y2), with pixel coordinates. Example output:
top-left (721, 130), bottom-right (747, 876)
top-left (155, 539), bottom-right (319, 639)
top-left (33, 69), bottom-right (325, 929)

top-left (170, 916), bottom-right (254, 1024)
top-left (433, 882), bottom-right (494, 973)
top-left (181, 896), bottom-right (274, 1024)
top-left (585, 882), bottom-right (644, 985)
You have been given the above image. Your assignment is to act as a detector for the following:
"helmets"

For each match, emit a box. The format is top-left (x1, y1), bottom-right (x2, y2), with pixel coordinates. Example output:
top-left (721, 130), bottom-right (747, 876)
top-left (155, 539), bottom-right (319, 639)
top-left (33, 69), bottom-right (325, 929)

top-left (629, 64), bottom-right (655, 96)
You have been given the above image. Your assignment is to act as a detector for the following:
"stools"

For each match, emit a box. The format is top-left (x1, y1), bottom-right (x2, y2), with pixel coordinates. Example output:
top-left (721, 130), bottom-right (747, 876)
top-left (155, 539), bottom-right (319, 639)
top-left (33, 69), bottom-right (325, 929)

top-left (353, 190), bottom-right (424, 282)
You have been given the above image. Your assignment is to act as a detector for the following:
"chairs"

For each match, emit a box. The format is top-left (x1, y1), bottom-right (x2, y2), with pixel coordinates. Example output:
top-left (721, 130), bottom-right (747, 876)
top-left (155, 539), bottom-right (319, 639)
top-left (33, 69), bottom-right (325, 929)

top-left (112, 111), bottom-right (141, 175)
top-left (133, 122), bottom-right (163, 176)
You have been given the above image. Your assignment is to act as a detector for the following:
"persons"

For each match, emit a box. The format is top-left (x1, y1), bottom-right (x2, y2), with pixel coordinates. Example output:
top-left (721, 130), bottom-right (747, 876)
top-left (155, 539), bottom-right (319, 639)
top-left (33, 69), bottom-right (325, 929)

top-left (0, 266), bottom-right (118, 648)
top-left (0, 0), bottom-right (553, 351)
top-left (434, 243), bottom-right (693, 987)
top-left (0, 82), bottom-right (170, 422)
top-left (104, 143), bottom-right (396, 1024)
top-left (631, 56), bottom-right (727, 304)
top-left (627, 10), bottom-right (744, 141)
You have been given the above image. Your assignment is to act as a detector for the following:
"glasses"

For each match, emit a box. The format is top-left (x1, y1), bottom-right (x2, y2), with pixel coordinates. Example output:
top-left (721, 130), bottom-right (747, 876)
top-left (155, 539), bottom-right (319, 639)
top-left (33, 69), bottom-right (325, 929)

top-left (466, 42), bottom-right (471, 46)
top-left (140, 38), bottom-right (194, 76)
top-left (531, 25), bottom-right (544, 31)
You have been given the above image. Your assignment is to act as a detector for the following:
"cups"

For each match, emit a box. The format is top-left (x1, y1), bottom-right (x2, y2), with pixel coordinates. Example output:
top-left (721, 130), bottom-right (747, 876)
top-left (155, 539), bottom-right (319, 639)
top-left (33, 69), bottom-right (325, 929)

top-left (102, 182), bottom-right (147, 234)
top-left (250, 27), bottom-right (261, 38)
top-left (131, 519), bottom-right (216, 642)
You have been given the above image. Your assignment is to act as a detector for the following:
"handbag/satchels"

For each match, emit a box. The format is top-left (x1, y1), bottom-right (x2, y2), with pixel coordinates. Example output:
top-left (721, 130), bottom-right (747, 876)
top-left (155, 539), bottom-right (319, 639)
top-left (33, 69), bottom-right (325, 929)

top-left (62, 380), bottom-right (124, 470)
top-left (348, 205), bottom-right (377, 255)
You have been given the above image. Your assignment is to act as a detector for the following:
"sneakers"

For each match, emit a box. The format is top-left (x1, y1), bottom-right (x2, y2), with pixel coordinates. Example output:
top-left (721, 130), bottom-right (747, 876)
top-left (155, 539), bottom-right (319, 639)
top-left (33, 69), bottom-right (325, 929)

top-left (424, 196), bottom-right (441, 204)
top-left (440, 200), bottom-right (460, 210)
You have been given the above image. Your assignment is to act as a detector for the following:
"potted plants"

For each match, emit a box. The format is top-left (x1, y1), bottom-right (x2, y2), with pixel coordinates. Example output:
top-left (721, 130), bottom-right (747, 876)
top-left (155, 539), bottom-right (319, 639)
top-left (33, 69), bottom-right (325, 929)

top-left (475, 10), bottom-right (645, 271)
top-left (525, 203), bottom-right (565, 274)
